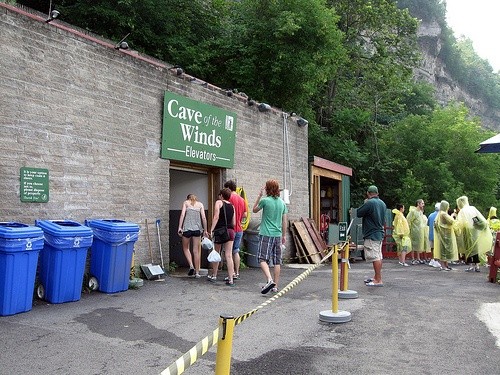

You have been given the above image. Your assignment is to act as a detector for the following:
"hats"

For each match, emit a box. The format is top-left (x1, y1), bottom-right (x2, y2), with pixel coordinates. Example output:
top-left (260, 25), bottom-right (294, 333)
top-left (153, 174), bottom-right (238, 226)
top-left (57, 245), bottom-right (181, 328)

top-left (368, 185), bottom-right (378, 193)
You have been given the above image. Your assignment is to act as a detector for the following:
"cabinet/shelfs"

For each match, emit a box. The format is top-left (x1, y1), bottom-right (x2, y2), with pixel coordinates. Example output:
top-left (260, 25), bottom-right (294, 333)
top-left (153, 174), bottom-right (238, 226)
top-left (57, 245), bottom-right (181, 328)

top-left (321, 184), bottom-right (338, 221)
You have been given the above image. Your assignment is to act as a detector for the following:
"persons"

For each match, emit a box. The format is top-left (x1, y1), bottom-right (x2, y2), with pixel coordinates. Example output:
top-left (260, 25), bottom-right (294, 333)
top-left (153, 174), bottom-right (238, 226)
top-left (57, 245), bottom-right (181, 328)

top-left (357, 185), bottom-right (386, 287)
top-left (253, 179), bottom-right (288, 294)
top-left (178, 194), bottom-right (208, 279)
top-left (392, 204), bottom-right (412, 267)
top-left (422, 196), bottom-right (500, 272)
top-left (406, 199), bottom-right (425, 265)
top-left (206, 180), bottom-right (246, 285)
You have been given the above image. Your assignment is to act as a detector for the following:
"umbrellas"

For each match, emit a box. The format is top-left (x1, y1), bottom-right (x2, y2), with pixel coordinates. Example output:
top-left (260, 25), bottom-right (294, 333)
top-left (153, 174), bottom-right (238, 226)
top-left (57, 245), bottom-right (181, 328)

top-left (474, 134), bottom-right (500, 153)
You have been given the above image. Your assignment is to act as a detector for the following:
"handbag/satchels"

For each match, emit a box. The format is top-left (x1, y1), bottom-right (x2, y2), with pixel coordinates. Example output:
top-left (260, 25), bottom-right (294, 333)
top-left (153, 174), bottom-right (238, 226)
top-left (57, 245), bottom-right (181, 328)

top-left (226, 228), bottom-right (234, 241)
top-left (201, 236), bottom-right (213, 250)
top-left (207, 243), bottom-right (221, 262)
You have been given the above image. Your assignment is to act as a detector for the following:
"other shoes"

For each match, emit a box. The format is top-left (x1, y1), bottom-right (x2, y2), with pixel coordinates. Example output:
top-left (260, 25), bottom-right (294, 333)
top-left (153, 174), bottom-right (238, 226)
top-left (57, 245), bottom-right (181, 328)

top-left (224, 273), bottom-right (237, 280)
top-left (207, 274), bottom-right (216, 280)
top-left (188, 267), bottom-right (201, 279)
top-left (399, 258), bottom-right (481, 272)
top-left (226, 280), bottom-right (234, 286)
top-left (236, 274), bottom-right (240, 279)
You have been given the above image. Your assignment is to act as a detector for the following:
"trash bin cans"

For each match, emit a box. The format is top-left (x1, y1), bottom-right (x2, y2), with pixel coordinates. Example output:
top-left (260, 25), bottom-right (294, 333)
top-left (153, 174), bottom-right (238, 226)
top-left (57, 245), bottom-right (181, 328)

top-left (36, 220), bottom-right (93, 304)
top-left (0, 222), bottom-right (44, 317)
top-left (89, 219), bottom-right (140, 293)
top-left (246, 229), bottom-right (278, 268)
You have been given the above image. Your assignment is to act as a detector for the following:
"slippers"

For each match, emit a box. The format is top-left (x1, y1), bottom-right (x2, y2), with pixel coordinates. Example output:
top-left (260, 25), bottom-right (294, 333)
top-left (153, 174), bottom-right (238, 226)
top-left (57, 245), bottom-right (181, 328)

top-left (364, 278), bottom-right (384, 287)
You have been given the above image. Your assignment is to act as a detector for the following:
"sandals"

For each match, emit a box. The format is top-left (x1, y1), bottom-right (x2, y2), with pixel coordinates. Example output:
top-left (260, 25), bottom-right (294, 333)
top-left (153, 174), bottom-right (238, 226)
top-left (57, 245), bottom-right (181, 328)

top-left (260, 279), bottom-right (278, 294)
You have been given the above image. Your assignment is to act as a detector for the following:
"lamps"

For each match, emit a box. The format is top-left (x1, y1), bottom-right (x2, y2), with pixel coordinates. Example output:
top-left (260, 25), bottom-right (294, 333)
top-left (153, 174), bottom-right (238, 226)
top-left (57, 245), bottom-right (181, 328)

top-left (291, 112), bottom-right (309, 125)
top-left (46, 10), bottom-right (60, 23)
top-left (115, 42), bottom-right (129, 50)
top-left (249, 99), bottom-right (272, 112)
top-left (169, 66), bottom-right (185, 76)
